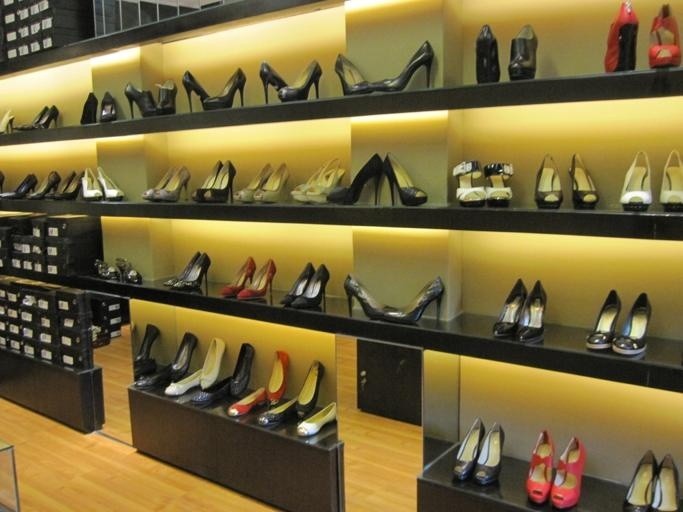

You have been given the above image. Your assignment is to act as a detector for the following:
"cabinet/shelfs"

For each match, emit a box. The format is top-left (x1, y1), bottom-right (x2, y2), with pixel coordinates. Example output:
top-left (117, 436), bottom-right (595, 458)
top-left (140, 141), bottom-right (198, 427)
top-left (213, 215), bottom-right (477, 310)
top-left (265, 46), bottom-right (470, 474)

top-left (0, 1), bottom-right (682, 399)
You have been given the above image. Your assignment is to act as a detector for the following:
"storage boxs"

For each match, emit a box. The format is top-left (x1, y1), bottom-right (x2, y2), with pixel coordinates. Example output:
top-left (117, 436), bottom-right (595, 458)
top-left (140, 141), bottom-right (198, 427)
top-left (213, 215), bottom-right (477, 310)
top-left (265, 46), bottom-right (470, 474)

top-left (0, 211), bottom-right (131, 368)
top-left (0, 1), bottom-right (97, 64)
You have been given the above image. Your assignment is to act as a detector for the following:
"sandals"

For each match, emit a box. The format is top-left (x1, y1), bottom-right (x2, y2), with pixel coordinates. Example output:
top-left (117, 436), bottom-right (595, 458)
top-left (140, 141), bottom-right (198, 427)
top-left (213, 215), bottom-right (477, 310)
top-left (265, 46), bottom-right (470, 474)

top-left (568, 154), bottom-right (599, 210)
top-left (535, 154), bottom-right (563, 209)
top-left (620, 151), bottom-right (653, 211)
top-left (660, 149), bottom-right (683, 212)
top-left (453, 160), bottom-right (514, 208)
top-left (454, 416), bottom-right (679, 512)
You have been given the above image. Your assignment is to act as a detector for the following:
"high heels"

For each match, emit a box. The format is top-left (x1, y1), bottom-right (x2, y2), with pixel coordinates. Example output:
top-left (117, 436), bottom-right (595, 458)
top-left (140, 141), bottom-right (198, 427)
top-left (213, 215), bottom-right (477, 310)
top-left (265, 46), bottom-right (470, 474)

top-left (260, 62), bottom-right (288, 104)
top-left (100, 91), bottom-right (116, 120)
top-left (183, 71), bottom-right (210, 112)
top-left (278, 60), bottom-right (322, 102)
top-left (156, 79), bottom-right (177, 113)
top-left (367, 40), bottom-right (434, 90)
top-left (142, 153), bottom-right (428, 207)
top-left (476, 25), bottom-right (500, 84)
top-left (203, 68), bottom-right (247, 108)
top-left (508, 25), bottom-right (537, 80)
top-left (162, 251), bottom-right (329, 310)
top-left (605, 1), bottom-right (639, 72)
top-left (0, 167), bottom-right (125, 201)
top-left (334, 53), bottom-right (372, 95)
top-left (125, 82), bottom-right (160, 118)
top-left (95, 259), bottom-right (118, 280)
top-left (648, 5), bottom-right (682, 69)
top-left (115, 256), bottom-right (141, 283)
top-left (80, 93), bottom-right (98, 124)
top-left (383, 276), bottom-right (444, 324)
top-left (343, 273), bottom-right (385, 321)
top-left (0, 105), bottom-right (58, 134)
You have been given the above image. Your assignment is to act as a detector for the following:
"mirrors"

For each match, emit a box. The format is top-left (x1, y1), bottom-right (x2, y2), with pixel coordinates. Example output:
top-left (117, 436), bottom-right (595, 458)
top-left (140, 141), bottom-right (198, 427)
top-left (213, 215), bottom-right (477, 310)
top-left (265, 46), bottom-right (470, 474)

top-left (334, 332), bottom-right (459, 512)
top-left (84, 289), bottom-right (134, 446)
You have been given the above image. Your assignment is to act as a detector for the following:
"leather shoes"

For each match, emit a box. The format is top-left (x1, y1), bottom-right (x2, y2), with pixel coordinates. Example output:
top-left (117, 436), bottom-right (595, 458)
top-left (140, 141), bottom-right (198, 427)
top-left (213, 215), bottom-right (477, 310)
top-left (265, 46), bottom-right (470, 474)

top-left (586, 291), bottom-right (620, 350)
top-left (133, 325), bottom-right (337, 437)
top-left (612, 293), bottom-right (651, 355)
top-left (493, 279), bottom-right (547, 342)
